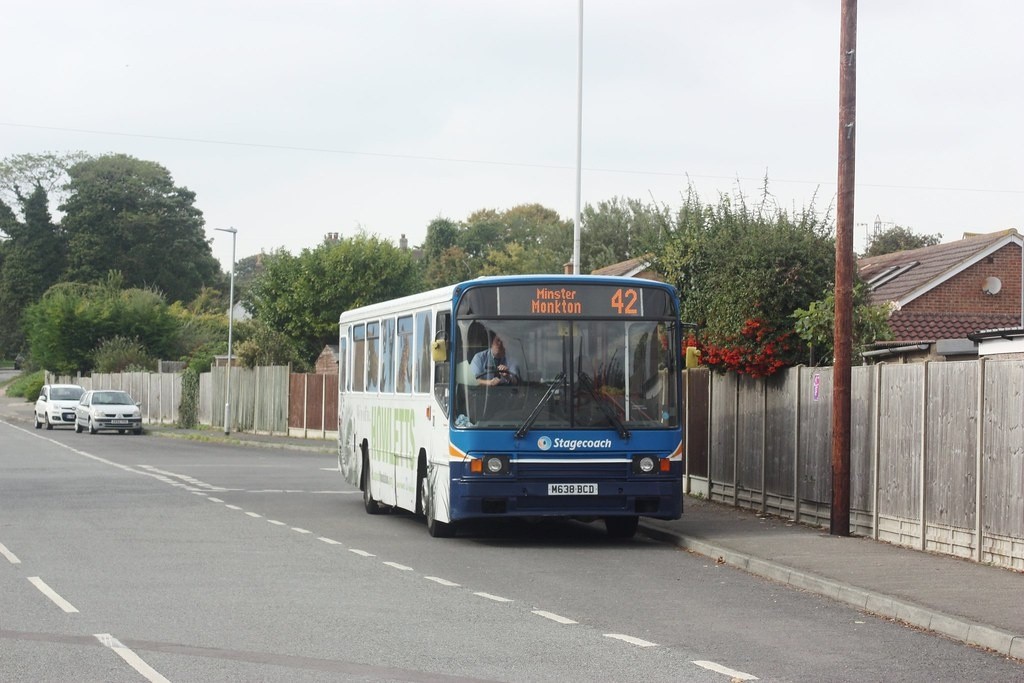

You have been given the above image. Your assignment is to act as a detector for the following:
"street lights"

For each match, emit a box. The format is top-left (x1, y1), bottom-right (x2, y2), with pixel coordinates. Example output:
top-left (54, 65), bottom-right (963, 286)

top-left (215, 227), bottom-right (238, 436)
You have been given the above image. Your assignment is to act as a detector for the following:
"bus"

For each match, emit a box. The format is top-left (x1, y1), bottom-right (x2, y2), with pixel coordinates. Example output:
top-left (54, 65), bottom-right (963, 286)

top-left (337, 275), bottom-right (684, 539)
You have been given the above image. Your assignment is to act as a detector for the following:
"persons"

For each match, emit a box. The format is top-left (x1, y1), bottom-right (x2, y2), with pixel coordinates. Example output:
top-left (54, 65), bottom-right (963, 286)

top-left (471, 330), bottom-right (532, 386)
top-left (108, 394), bottom-right (122, 403)
top-left (575, 355), bottom-right (594, 378)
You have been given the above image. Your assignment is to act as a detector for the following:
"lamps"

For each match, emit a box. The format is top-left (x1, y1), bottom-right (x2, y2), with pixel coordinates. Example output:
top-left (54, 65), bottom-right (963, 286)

top-left (981, 276), bottom-right (1002, 296)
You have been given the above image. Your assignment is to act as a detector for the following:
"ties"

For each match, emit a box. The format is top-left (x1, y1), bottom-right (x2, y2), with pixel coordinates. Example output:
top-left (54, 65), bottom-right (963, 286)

top-left (493, 358), bottom-right (501, 379)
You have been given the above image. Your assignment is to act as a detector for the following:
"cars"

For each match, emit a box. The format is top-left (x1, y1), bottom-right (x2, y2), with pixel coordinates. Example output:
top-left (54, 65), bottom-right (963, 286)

top-left (14, 355), bottom-right (24, 369)
top-left (74, 390), bottom-right (142, 435)
top-left (34, 383), bottom-right (87, 430)
